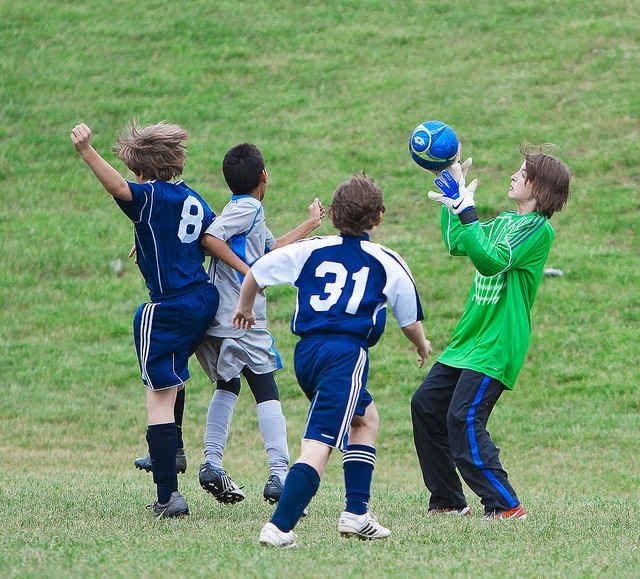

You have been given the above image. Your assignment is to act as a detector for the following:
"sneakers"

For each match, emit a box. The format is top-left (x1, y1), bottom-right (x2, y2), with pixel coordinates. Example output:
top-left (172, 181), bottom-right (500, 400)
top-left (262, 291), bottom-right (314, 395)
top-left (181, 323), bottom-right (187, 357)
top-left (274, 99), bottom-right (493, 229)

top-left (338, 507), bottom-right (391, 540)
top-left (481, 505), bottom-right (526, 521)
top-left (134, 448), bottom-right (187, 474)
top-left (259, 521), bottom-right (298, 549)
top-left (198, 463), bottom-right (245, 505)
top-left (425, 508), bottom-right (471, 518)
top-left (145, 491), bottom-right (188, 518)
top-left (264, 474), bottom-right (308, 517)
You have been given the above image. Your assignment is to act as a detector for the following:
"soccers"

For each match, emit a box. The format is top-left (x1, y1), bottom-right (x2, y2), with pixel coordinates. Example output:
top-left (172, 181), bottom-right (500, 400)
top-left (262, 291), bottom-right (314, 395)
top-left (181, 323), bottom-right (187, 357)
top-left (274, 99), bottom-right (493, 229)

top-left (408, 120), bottom-right (459, 170)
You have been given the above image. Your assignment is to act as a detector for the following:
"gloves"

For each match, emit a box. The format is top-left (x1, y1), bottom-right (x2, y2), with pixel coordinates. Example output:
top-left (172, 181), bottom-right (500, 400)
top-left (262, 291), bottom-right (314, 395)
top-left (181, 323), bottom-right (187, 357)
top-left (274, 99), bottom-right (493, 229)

top-left (428, 163), bottom-right (478, 215)
top-left (431, 142), bottom-right (472, 185)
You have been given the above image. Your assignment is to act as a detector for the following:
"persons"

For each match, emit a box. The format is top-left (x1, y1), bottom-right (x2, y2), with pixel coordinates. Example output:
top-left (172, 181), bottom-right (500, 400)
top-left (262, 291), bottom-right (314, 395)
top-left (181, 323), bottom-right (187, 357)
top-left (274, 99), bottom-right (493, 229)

top-left (409, 140), bottom-right (572, 522)
top-left (67, 114), bottom-right (219, 518)
top-left (197, 142), bottom-right (328, 517)
top-left (231, 168), bottom-right (433, 552)
